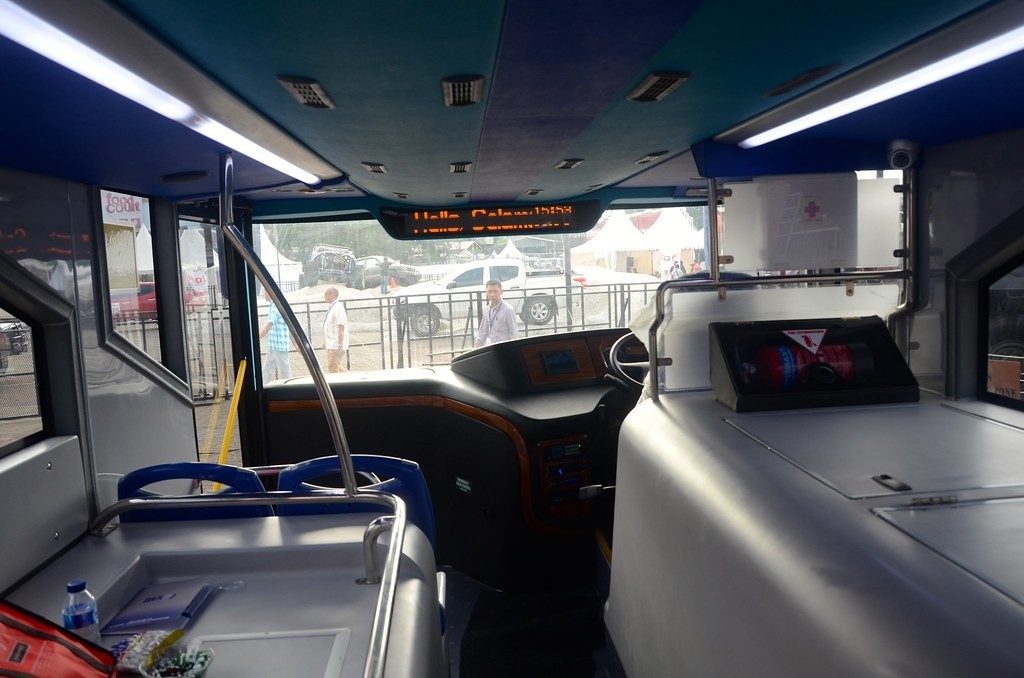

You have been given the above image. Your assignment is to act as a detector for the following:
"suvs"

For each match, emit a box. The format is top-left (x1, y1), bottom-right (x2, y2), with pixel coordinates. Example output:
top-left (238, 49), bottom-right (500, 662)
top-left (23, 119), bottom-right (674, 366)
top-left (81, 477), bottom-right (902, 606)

top-left (109, 283), bottom-right (158, 324)
top-left (0, 321), bottom-right (28, 355)
top-left (299, 244), bottom-right (359, 288)
top-left (354, 256), bottom-right (422, 289)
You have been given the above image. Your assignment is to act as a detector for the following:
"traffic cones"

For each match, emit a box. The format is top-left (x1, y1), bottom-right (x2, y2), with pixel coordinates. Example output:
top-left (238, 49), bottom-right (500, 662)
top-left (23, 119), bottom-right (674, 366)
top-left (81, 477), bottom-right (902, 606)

top-left (389, 276), bottom-right (397, 289)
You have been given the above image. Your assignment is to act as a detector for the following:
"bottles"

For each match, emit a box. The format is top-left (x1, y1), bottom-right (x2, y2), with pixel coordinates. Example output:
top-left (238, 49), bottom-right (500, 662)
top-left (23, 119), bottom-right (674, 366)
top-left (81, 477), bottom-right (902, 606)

top-left (60, 579), bottom-right (102, 647)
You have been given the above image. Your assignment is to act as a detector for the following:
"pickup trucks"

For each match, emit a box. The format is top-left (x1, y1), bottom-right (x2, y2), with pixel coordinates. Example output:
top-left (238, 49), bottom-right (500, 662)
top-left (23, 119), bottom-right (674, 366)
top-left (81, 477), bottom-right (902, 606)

top-left (388, 258), bottom-right (587, 337)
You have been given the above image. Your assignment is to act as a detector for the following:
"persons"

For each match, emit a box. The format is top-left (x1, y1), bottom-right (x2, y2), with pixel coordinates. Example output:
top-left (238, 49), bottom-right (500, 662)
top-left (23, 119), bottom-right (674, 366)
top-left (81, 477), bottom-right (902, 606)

top-left (323, 287), bottom-right (349, 373)
top-left (259, 287), bottom-right (300, 384)
top-left (380, 257), bottom-right (395, 294)
top-left (693, 259), bottom-right (706, 272)
top-left (474, 279), bottom-right (518, 348)
top-left (669, 260), bottom-right (686, 279)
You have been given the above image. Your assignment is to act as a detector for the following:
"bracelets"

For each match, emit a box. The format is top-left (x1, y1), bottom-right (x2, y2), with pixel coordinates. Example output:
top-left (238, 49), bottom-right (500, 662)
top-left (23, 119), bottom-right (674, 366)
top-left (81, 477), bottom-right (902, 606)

top-left (338, 344), bottom-right (343, 348)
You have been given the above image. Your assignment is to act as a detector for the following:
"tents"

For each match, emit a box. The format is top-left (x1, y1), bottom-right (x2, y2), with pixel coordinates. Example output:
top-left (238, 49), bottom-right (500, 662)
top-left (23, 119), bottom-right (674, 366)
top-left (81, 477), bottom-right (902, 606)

top-left (570, 207), bottom-right (704, 272)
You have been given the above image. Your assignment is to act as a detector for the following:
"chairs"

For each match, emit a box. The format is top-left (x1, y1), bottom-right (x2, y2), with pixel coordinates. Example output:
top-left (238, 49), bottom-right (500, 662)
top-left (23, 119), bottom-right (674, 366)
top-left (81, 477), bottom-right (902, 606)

top-left (117, 454), bottom-right (447, 634)
top-left (678, 272), bottom-right (754, 290)
